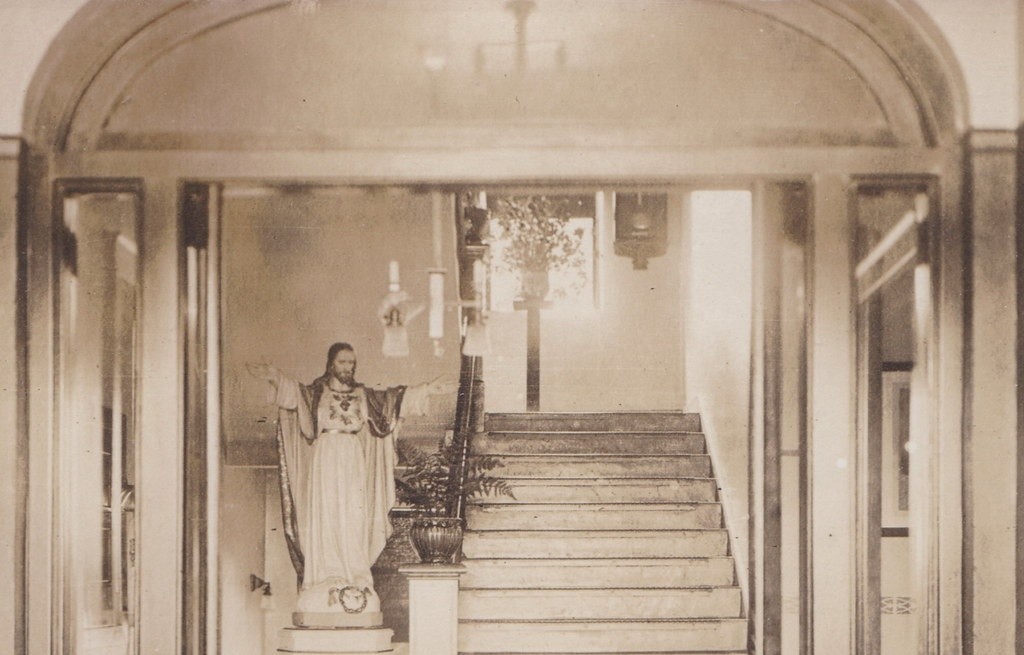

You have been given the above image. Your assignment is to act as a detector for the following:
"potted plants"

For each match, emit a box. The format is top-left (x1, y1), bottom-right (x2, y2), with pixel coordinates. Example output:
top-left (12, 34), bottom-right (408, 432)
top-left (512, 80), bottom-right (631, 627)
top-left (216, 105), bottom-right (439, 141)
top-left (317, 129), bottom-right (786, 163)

top-left (395, 417), bottom-right (515, 563)
top-left (495, 191), bottom-right (590, 298)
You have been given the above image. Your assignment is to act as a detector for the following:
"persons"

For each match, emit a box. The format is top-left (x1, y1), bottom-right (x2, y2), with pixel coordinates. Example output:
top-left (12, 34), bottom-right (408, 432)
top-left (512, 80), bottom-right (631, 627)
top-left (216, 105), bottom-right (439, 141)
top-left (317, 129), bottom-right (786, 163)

top-left (246, 342), bottom-right (461, 613)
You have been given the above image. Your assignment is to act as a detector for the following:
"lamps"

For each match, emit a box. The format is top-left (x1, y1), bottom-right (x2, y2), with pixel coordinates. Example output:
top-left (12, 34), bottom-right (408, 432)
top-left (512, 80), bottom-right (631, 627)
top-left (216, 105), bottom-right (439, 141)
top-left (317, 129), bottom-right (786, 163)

top-left (427, 192), bottom-right (447, 355)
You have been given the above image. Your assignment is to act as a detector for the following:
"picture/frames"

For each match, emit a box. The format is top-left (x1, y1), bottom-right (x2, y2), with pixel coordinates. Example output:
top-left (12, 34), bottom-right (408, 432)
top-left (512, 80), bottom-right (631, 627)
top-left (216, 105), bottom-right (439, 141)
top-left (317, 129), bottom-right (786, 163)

top-left (881, 359), bottom-right (913, 538)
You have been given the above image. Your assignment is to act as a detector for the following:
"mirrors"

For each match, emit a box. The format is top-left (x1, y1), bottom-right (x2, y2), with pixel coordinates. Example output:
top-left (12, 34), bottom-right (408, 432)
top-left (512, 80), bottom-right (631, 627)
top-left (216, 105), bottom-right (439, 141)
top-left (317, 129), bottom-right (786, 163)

top-left (50, 178), bottom-right (141, 655)
